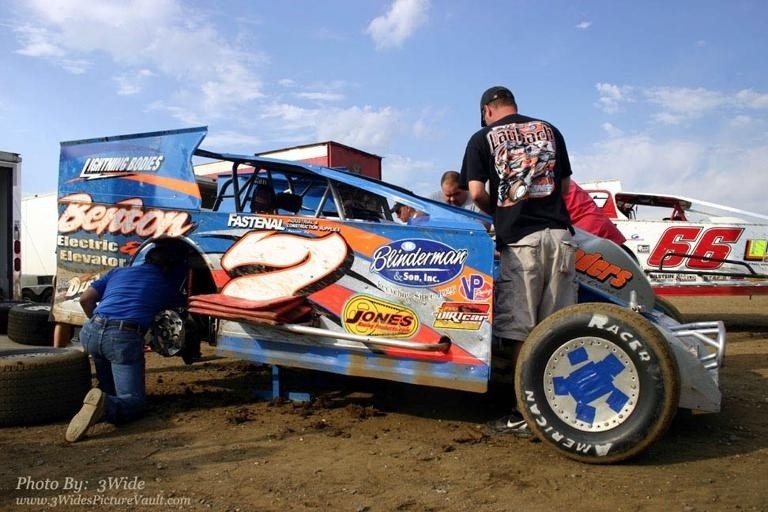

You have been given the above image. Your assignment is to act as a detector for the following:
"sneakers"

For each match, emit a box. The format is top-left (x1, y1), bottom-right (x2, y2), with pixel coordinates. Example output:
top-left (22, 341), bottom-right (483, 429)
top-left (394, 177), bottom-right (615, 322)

top-left (486, 408), bottom-right (535, 436)
top-left (65, 387), bottom-right (106, 442)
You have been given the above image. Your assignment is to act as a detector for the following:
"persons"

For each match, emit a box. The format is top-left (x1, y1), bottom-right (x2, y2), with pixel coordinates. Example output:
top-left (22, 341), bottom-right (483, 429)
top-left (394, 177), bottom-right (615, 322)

top-left (48, 247), bottom-right (73, 349)
top-left (63, 245), bottom-right (196, 444)
top-left (456, 85), bottom-right (579, 441)
top-left (558, 175), bottom-right (627, 246)
top-left (427, 170), bottom-right (491, 233)
top-left (390, 198), bottom-right (424, 225)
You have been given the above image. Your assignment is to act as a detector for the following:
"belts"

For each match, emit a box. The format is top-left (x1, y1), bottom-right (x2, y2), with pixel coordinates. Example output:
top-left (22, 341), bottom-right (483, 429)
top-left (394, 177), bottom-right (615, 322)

top-left (93, 315), bottom-right (148, 334)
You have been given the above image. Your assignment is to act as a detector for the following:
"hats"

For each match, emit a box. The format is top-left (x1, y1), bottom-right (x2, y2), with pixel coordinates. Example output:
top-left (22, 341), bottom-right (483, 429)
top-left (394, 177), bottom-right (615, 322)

top-left (479, 85), bottom-right (515, 128)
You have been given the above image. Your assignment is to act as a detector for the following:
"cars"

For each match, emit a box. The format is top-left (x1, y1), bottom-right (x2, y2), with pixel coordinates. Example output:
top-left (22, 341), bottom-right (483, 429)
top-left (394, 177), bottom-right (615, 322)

top-left (48, 125), bottom-right (768, 465)
